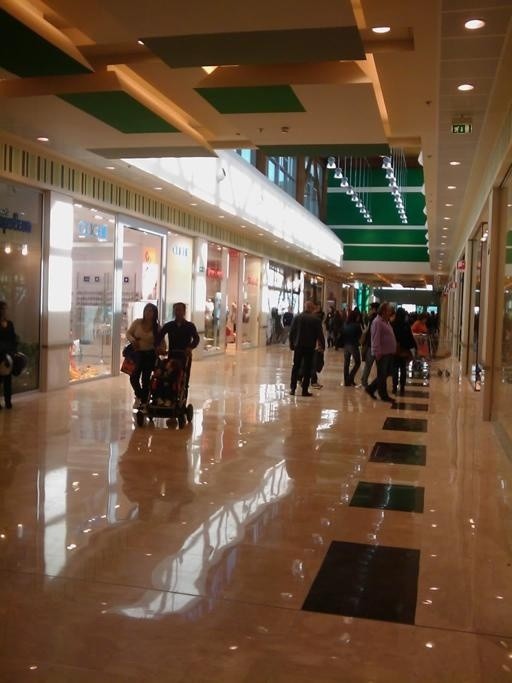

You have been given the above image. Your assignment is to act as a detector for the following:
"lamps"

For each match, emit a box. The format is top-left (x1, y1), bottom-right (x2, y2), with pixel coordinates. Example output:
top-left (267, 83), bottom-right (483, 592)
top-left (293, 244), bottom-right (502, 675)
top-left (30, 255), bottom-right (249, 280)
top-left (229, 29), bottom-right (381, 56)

top-left (327, 153), bottom-right (373, 223)
top-left (382, 145), bottom-right (409, 225)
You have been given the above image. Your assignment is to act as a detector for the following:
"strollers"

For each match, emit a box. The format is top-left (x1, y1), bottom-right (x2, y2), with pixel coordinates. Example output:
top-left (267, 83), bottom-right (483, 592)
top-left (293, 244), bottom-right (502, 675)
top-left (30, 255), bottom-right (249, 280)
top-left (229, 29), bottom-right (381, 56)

top-left (135, 350), bottom-right (194, 430)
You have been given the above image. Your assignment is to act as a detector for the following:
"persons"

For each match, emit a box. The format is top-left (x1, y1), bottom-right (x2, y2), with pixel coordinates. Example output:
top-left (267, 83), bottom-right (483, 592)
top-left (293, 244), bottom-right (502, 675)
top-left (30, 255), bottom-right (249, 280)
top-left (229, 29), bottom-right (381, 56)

top-left (157, 358), bottom-right (179, 407)
top-left (126, 303), bottom-right (166, 409)
top-left (272, 299), bottom-right (440, 401)
top-left (0, 301), bottom-right (18, 409)
top-left (152, 302), bottom-right (200, 387)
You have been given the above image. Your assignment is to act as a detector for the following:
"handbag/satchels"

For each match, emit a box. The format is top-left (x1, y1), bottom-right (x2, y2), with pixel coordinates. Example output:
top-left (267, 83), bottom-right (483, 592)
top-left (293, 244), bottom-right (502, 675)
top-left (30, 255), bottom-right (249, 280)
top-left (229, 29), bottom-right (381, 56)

top-left (396, 343), bottom-right (412, 361)
top-left (8, 352), bottom-right (26, 375)
top-left (121, 358), bottom-right (136, 376)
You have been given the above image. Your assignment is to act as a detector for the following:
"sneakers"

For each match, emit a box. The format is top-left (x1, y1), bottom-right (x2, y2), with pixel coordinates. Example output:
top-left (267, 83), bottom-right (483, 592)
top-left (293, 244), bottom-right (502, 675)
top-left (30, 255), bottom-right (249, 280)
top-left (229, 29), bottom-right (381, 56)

top-left (159, 398), bottom-right (171, 406)
top-left (133, 398), bottom-right (141, 409)
top-left (290, 379), bottom-right (322, 396)
top-left (344, 375), bottom-right (404, 402)
top-left (166, 419), bottom-right (177, 424)
top-left (5, 400), bottom-right (12, 409)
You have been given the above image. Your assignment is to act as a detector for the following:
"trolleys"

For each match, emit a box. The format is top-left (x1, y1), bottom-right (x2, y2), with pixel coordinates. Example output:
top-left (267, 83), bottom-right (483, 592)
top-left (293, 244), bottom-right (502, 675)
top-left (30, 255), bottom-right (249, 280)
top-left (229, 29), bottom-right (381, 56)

top-left (412, 333), bottom-right (453, 379)
